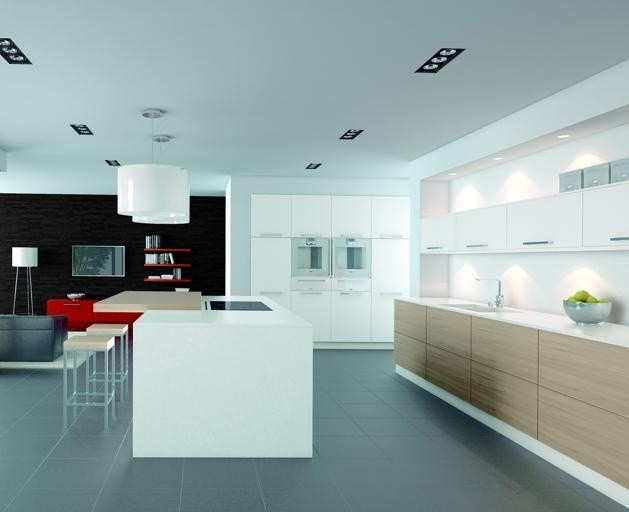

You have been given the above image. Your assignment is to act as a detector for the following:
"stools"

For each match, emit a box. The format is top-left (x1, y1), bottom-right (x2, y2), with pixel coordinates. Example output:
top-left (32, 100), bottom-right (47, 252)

top-left (61, 335), bottom-right (116, 428)
top-left (86, 323), bottom-right (129, 403)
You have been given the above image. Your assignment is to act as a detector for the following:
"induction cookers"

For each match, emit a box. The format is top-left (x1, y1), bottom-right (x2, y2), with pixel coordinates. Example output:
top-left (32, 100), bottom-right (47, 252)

top-left (210, 301), bottom-right (272, 310)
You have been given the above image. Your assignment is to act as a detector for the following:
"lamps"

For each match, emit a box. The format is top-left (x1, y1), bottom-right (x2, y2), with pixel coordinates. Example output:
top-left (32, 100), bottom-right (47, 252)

top-left (132, 136), bottom-right (190, 225)
top-left (12, 247), bottom-right (38, 317)
top-left (118, 109), bottom-right (181, 216)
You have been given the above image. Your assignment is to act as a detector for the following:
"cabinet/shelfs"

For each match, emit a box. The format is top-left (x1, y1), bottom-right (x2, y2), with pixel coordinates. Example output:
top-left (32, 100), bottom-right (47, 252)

top-left (394, 300), bottom-right (629, 490)
top-left (250, 195), bottom-right (410, 349)
top-left (144, 248), bottom-right (192, 282)
top-left (419, 181), bottom-right (629, 254)
top-left (47, 299), bottom-right (142, 330)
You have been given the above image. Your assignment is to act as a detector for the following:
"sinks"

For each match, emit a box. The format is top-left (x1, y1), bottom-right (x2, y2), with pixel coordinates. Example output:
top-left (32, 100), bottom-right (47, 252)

top-left (436, 297), bottom-right (517, 314)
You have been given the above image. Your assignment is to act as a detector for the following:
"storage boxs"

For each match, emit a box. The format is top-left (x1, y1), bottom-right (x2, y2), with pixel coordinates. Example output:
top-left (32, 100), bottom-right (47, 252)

top-left (560, 159), bottom-right (629, 191)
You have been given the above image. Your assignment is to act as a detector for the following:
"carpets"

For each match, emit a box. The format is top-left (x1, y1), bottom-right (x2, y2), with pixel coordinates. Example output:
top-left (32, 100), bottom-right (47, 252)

top-left (0, 331), bottom-right (93, 369)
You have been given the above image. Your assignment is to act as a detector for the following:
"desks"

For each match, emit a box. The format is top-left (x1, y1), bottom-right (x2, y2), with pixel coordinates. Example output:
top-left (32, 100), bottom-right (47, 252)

top-left (93, 289), bottom-right (202, 313)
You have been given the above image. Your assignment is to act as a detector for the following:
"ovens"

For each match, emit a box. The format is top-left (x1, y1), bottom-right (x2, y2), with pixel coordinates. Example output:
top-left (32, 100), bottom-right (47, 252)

top-left (330, 237), bottom-right (372, 279)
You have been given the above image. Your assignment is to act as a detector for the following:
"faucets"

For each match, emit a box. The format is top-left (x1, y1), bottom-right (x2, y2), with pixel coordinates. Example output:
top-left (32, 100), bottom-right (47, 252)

top-left (476, 275), bottom-right (504, 309)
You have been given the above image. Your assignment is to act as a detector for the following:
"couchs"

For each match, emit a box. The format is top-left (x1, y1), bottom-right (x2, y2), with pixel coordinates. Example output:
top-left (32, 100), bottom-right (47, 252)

top-left (0, 313), bottom-right (67, 361)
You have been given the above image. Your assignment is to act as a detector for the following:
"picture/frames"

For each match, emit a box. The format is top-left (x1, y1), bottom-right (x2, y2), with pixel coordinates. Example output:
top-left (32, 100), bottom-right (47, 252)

top-left (72, 245), bottom-right (124, 278)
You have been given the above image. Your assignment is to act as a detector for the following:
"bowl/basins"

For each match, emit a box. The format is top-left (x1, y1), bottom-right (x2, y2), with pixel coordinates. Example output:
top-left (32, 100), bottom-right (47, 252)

top-left (563, 300), bottom-right (613, 328)
top-left (66, 293), bottom-right (85, 299)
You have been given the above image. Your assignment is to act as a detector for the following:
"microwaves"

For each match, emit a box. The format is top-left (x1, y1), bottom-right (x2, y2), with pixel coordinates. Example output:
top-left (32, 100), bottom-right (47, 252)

top-left (292, 237), bottom-right (330, 276)
